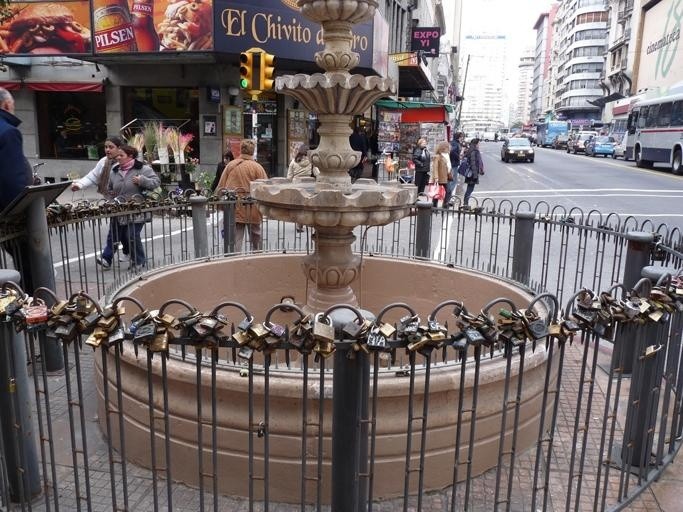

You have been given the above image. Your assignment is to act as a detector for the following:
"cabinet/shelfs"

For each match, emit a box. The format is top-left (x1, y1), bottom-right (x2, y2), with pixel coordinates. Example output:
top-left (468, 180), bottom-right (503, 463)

top-left (377, 122), bottom-right (400, 153)
top-left (400, 124), bottom-right (420, 153)
top-left (421, 124), bottom-right (445, 154)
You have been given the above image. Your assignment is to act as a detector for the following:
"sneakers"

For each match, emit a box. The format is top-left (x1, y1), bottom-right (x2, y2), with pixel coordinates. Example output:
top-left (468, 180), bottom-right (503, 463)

top-left (96, 258), bottom-right (110, 270)
top-left (119, 254), bottom-right (146, 273)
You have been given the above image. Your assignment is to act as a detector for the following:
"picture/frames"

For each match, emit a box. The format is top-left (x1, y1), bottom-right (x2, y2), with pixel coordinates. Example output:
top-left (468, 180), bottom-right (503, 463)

top-left (289, 107), bottom-right (309, 139)
top-left (288, 141), bottom-right (311, 167)
top-left (221, 104), bottom-right (244, 135)
top-left (223, 136), bottom-right (246, 161)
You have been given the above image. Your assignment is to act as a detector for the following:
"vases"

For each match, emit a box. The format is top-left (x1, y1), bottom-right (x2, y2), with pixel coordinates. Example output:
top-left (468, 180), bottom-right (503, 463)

top-left (173, 151), bottom-right (185, 164)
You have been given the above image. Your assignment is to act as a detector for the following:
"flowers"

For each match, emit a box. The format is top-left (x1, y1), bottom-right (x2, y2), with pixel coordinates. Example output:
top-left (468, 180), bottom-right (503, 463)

top-left (169, 127), bottom-right (195, 153)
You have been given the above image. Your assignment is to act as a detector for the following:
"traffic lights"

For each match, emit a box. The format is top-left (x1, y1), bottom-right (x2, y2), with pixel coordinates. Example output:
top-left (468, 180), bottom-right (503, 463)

top-left (259, 51), bottom-right (276, 92)
top-left (238, 51), bottom-right (253, 91)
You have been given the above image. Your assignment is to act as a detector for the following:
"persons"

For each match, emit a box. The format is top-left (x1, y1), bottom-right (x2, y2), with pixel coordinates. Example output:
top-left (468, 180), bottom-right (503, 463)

top-left (432, 141), bottom-right (454, 209)
top-left (107, 144), bottom-right (161, 274)
top-left (211, 150), bottom-right (234, 238)
top-left (0, 87), bottom-right (35, 298)
top-left (214, 138), bottom-right (268, 253)
top-left (70, 135), bottom-right (131, 271)
top-left (463, 138), bottom-right (484, 211)
top-left (449, 132), bottom-right (464, 194)
top-left (412, 138), bottom-right (430, 197)
top-left (287, 145), bottom-right (321, 238)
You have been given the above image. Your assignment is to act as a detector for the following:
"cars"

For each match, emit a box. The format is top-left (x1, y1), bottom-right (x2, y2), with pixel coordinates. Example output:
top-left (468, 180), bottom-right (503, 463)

top-left (475, 116), bottom-right (632, 161)
top-left (499, 135), bottom-right (536, 163)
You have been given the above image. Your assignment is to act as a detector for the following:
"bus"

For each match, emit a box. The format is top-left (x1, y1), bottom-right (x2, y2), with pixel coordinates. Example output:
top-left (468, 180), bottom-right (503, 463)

top-left (623, 91), bottom-right (682, 176)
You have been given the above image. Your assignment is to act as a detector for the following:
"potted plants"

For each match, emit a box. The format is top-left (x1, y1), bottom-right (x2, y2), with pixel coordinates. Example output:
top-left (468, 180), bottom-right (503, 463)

top-left (122, 127), bottom-right (144, 164)
top-left (142, 125), bottom-right (154, 167)
top-left (154, 122), bottom-right (169, 166)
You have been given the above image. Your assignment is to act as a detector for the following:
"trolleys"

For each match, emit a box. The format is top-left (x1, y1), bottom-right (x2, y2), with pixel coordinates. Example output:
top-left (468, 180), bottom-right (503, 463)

top-left (396, 168), bottom-right (415, 186)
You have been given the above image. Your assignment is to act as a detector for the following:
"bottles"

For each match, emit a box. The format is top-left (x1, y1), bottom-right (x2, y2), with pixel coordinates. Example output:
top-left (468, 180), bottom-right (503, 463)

top-left (93, 0), bottom-right (136, 53)
top-left (128, 1), bottom-right (161, 53)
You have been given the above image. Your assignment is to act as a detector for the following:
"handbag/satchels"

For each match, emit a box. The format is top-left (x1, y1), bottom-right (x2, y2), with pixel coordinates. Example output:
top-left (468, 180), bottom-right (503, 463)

top-left (423, 181), bottom-right (446, 200)
top-left (137, 164), bottom-right (163, 204)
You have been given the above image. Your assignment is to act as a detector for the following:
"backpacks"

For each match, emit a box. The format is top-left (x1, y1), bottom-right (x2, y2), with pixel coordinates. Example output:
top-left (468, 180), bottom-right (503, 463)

top-left (458, 149), bottom-right (476, 178)
